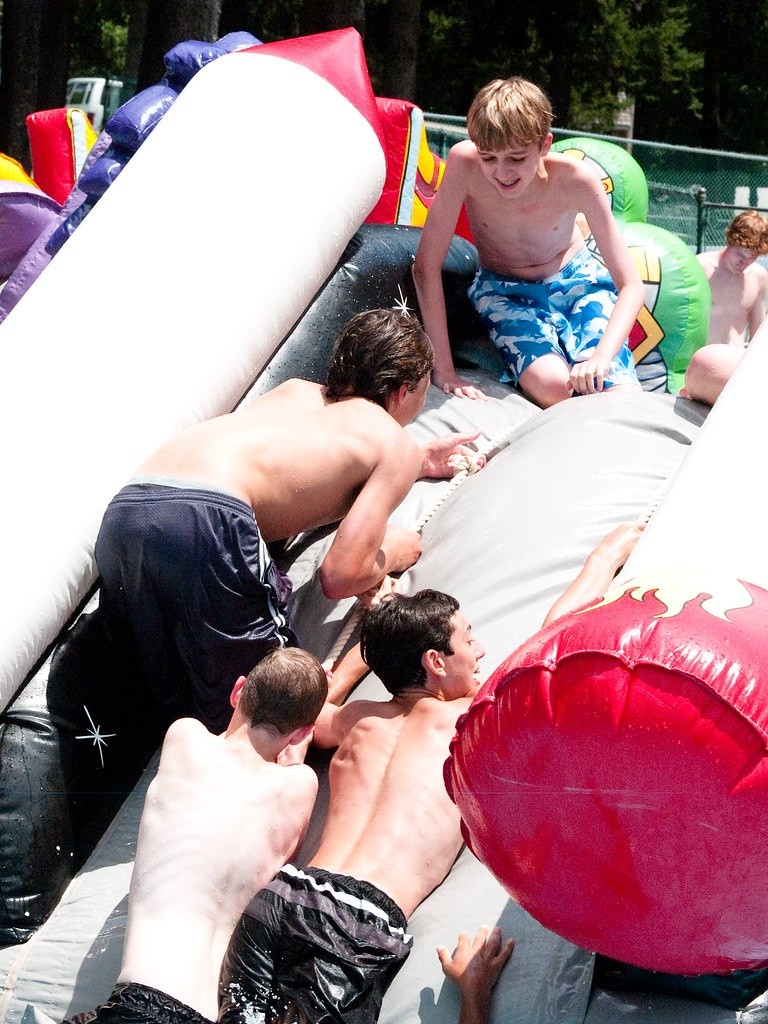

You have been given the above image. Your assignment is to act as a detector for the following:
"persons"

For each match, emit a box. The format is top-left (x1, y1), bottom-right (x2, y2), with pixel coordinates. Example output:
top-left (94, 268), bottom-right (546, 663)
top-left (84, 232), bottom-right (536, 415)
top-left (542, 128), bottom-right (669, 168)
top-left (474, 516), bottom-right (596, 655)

top-left (412, 75), bottom-right (647, 408)
top-left (60, 645), bottom-right (335, 1024)
top-left (695, 209), bottom-right (768, 348)
top-left (684, 343), bottom-right (748, 406)
top-left (216, 521), bottom-right (648, 1024)
top-left (93, 309), bottom-right (488, 735)
top-left (435, 926), bottom-right (518, 1024)
